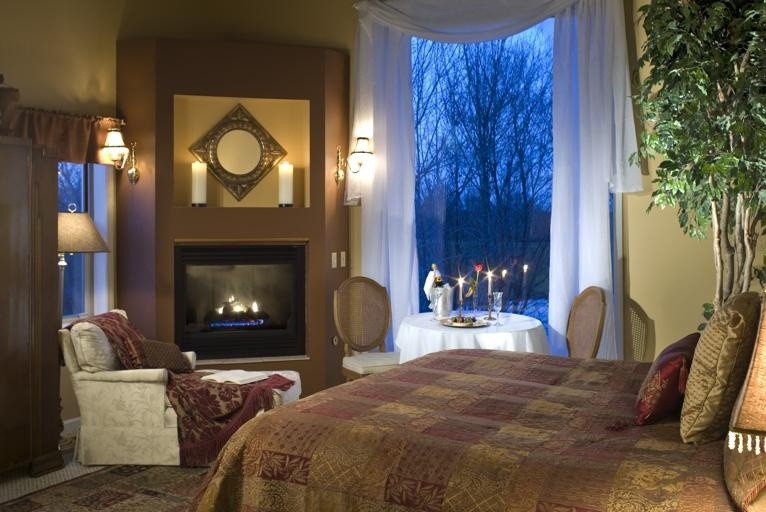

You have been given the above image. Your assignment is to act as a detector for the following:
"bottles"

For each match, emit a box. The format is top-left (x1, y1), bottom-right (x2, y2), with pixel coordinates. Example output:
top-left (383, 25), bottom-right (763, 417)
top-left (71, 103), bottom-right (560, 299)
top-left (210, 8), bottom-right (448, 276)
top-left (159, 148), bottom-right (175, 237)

top-left (431, 263), bottom-right (443, 286)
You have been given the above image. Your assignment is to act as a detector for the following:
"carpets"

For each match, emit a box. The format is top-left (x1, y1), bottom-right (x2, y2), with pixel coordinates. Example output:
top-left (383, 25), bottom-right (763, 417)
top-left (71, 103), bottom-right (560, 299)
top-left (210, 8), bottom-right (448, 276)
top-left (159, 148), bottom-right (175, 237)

top-left (0, 466), bottom-right (210, 511)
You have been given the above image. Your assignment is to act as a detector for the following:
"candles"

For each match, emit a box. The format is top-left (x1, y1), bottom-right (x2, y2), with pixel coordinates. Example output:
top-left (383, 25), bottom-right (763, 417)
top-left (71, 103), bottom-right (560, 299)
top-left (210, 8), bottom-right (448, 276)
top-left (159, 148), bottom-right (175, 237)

top-left (488, 272), bottom-right (492, 295)
top-left (275, 160), bottom-right (294, 203)
top-left (190, 161), bottom-right (211, 203)
top-left (458, 279), bottom-right (464, 300)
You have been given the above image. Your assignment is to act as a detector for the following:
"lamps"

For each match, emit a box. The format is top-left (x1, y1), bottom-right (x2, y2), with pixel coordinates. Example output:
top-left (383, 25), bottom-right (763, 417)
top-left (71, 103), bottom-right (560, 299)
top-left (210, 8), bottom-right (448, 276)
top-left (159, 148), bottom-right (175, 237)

top-left (99, 123), bottom-right (139, 185)
top-left (334, 138), bottom-right (376, 190)
top-left (51, 209), bottom-right (111, 327)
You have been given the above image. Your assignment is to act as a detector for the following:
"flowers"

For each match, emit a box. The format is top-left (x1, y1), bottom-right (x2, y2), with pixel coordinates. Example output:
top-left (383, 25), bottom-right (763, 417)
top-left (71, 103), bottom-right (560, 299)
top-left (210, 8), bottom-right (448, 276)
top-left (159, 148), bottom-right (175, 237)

top-left (471, 261), bottom-right (482, 290)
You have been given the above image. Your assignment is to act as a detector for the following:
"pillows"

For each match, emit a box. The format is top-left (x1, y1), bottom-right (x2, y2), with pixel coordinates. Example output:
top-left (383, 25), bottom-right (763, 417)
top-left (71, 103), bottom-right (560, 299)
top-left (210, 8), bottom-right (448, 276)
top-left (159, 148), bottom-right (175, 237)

top-left (682, 287), bottom-right (758, 447)
top-left (634, 331), bottom-right (705, 431)
top-left (721, 302), bottom-right (764, 511)
top-left (135, 334), bottom-right (193, 373)
top-left (67, 321), bottom-right (121, 371)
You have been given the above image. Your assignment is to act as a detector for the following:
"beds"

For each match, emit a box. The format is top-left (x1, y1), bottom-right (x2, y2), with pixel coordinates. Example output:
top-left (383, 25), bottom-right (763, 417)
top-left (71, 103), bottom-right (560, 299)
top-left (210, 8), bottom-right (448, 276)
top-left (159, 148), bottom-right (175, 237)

top-left (194, 264), bottom-right (765, 512)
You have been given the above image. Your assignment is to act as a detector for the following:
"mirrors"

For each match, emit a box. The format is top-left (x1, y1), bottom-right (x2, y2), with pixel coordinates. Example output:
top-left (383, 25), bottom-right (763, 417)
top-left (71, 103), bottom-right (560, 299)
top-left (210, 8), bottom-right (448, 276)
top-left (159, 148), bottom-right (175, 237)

top-left (186, 101), bottom-right (289, 200)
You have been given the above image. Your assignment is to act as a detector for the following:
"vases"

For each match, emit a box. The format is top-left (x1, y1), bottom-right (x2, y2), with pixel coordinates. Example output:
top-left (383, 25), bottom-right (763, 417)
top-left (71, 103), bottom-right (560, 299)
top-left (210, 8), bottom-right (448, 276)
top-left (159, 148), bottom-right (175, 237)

top-left (473, 288), bottom-right (477, 312)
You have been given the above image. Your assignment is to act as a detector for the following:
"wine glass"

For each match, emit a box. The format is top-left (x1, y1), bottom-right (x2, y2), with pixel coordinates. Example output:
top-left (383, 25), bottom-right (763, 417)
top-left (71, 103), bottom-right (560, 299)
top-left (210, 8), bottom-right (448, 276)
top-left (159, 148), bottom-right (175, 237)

top-left (493, 292), bottom-right (504, 325)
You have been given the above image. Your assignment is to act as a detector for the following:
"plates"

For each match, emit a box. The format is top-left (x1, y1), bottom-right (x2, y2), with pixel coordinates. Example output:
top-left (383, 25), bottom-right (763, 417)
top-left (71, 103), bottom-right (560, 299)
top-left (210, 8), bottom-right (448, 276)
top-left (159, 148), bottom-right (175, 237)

top-left (440, 318), bottom-right (487, 327)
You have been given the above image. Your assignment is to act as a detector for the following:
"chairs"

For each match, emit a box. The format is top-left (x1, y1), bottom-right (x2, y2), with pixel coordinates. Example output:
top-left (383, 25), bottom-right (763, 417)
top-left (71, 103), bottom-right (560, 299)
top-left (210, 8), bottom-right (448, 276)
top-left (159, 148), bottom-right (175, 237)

top-left (52, 309), bottom-right (304, 469)
top-left (566, 285), bottom-right (610, 358)
top-left (330, 274), bottom-right (403, 379)
